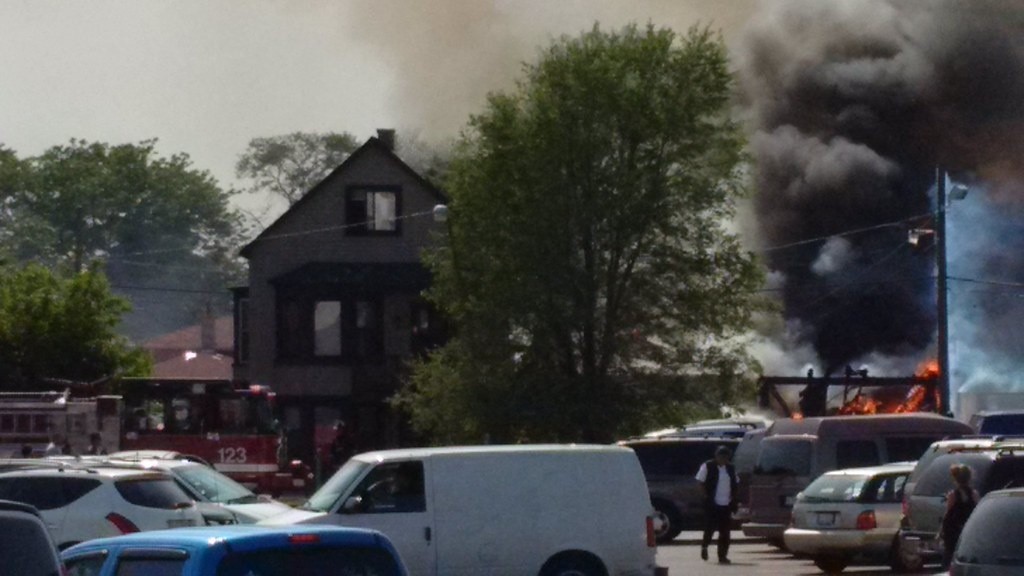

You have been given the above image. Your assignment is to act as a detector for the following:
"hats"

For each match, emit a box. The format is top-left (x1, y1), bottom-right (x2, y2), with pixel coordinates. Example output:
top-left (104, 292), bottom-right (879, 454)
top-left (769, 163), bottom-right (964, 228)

top-left (714, 445), bottom-right (730, 454)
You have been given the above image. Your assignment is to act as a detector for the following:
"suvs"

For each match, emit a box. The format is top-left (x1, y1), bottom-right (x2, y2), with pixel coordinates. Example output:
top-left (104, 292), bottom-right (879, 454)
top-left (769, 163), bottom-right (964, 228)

top-left (784, 410), bottom-right (1024, 576)
top-left (616, 419), bottom-right (766, 543)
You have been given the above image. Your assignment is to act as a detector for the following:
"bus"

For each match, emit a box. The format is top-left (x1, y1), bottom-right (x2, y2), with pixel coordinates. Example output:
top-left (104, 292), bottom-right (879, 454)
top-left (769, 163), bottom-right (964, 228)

top-left (120, 377), bottom-right (285, 483)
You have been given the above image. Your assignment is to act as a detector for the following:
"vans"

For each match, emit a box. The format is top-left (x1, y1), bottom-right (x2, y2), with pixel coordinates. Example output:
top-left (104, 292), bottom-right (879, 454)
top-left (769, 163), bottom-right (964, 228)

top-left (743, 413), bottom-right (977, 551)
top-left (256, 445), bottom-right (670, 576)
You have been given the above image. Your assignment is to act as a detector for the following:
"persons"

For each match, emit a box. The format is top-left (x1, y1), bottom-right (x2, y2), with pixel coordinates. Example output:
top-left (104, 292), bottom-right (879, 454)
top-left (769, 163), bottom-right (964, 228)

top-left (695, 446), bottom-right (740, 564)
top-left (87, 433), bottom-right (102, 455)
top-left (366, 462), bottom-right (425, 513)
top-left (21, 446), bottom-right (32, 458)
top-left (935, 464), bottom-right (979, 571)
top-left (46, 432), bottom-right (65, 456)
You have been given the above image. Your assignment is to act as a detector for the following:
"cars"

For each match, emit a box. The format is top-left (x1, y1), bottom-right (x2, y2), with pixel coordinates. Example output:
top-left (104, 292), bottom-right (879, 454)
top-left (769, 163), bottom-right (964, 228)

top-left (0, 450), bottom-right (411, 575)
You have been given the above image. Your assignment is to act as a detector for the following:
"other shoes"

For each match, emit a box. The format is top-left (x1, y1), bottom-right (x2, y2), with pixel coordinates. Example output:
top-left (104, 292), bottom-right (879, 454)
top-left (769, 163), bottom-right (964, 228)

top-left (718, 555), bottom-right (730, 563)
top-left (700, 547), bottom-right (708, 560)
